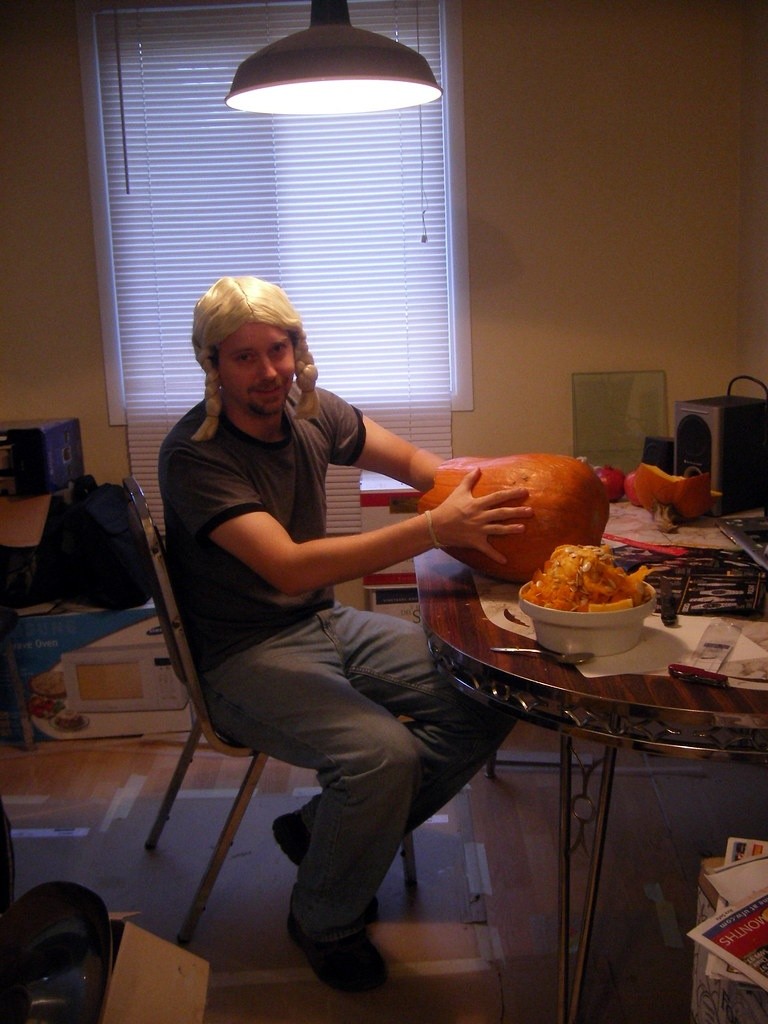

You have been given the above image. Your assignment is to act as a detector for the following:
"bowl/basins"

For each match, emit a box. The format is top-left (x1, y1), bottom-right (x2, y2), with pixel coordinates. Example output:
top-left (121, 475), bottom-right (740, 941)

top-left (518, 575), bottom-right (656, 656)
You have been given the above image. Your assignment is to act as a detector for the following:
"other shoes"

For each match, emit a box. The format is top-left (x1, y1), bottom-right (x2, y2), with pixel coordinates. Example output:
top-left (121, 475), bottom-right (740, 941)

top-left (287, 907), bottom-right (387, 992)
top-left (273, 812), bottom-right (380, 922)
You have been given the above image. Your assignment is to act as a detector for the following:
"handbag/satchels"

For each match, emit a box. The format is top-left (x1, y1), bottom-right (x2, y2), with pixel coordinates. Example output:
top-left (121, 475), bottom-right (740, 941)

top-left (34, 473), bottom-right (154, 607)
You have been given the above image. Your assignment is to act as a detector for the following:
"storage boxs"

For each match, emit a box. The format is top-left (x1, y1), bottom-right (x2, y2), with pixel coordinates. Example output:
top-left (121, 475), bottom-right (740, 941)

top-left (0, 418), bottom-right (84, 496)
top-left (4, 604), bottom-right (200, 745)
top-left (692, 856), bottom-right (768, 1024)
top-left (102, 910), bottom-right (211, 1023)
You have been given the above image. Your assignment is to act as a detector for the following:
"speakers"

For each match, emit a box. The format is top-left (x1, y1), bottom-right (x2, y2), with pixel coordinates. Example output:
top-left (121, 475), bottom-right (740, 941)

top-left (674, 395), bottom-right (768, 517)
top-left (642, 435), bottom-right (675, 475)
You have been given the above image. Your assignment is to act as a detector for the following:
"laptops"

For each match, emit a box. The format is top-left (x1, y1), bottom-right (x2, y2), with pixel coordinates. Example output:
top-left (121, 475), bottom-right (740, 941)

top-left (714, 515), bottom-right (768, 571)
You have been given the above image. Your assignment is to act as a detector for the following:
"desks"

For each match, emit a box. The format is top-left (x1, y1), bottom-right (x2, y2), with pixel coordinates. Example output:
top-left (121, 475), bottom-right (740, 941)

top-left (417, 536), bottom-right (767, 1024)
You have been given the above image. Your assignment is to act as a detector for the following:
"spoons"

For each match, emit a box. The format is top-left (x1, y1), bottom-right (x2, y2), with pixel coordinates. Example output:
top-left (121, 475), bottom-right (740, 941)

top-left (490, 647), bottom-right (595, 665)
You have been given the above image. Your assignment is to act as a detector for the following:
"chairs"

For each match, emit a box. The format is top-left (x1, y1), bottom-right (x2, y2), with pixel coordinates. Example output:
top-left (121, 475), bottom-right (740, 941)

top-left (123, 477), bottom-right (417, 942)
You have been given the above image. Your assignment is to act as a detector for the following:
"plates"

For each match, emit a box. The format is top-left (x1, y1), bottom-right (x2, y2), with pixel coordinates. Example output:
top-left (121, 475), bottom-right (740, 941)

top-left (49, 715), bottom-right (89, 733)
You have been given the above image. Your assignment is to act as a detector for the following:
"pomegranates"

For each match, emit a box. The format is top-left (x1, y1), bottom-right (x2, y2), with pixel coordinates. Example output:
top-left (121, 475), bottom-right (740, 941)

top-left (593, 464), bottom-right (643, 508)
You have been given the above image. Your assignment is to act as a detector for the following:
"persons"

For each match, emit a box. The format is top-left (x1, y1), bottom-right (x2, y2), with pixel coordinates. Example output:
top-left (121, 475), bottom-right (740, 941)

top-left (155, 275), bottom-right (533, 995)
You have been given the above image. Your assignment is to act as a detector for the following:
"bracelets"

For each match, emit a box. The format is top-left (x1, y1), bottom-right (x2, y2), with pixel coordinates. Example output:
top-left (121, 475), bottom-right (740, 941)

top-left (425, 511), bottom-right (441, 549)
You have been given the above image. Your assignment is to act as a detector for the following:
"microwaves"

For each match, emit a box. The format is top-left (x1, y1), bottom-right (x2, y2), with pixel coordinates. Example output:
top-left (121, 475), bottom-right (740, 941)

top-left (60, 641), bottom-right (191, 710)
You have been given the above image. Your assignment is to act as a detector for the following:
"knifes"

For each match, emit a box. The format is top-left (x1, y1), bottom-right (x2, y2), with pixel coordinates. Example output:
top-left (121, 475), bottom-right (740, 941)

top-left (668, 664), bottom-right (768, 691)
top-left (660, 578), bottom-right (677, 626)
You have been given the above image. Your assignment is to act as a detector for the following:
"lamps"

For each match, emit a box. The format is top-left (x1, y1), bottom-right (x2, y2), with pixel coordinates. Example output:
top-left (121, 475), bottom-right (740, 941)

top-left (224, 0), bottom-right (445, 117)
